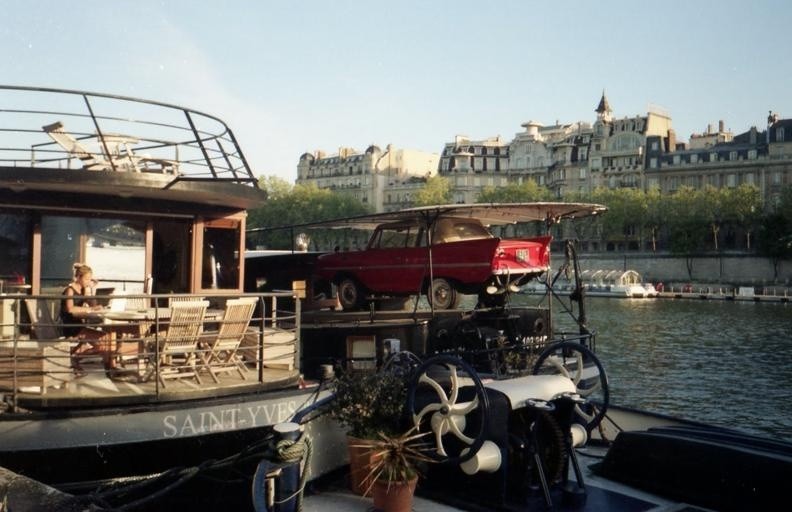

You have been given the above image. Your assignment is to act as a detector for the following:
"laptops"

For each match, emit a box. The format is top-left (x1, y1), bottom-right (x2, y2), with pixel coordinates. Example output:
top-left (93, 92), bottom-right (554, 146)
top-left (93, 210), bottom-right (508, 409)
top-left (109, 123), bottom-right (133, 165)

top-left (95, 288), bottom-right (115, 308)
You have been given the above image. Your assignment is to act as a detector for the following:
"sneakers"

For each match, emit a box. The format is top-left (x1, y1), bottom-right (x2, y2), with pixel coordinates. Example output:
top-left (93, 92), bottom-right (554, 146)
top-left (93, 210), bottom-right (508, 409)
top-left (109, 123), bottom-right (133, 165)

top-left (106, 366), bottom-right (130, 377)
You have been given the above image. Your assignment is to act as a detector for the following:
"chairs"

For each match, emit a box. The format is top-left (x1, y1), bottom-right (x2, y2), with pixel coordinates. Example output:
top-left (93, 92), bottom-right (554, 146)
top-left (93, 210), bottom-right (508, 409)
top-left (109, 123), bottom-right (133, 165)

top-left (42, 121), bottom-right (181, 174)
top-left (25, 290), bottom-right (258, 389)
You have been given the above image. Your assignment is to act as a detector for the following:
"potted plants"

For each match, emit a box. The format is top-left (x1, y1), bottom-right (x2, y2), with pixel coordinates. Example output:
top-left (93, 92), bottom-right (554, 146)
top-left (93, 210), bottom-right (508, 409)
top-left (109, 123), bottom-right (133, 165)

top-left (310, 358), bottom-right (445, 511)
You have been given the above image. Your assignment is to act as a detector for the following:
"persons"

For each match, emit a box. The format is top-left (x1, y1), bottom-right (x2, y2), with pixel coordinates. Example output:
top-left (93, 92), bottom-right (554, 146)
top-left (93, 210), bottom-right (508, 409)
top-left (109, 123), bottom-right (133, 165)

top-left (56, 261), bottom-right (119, 379)
